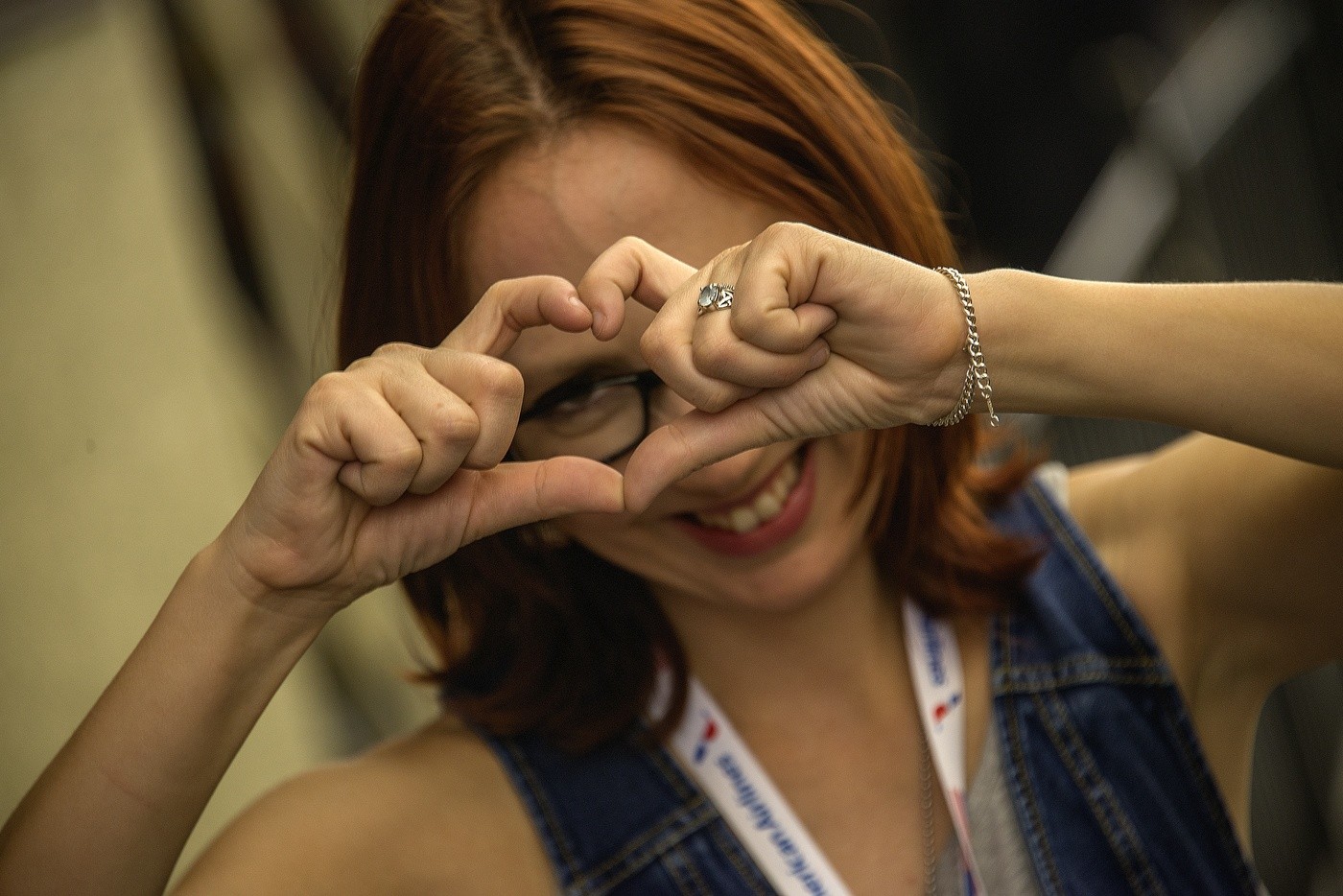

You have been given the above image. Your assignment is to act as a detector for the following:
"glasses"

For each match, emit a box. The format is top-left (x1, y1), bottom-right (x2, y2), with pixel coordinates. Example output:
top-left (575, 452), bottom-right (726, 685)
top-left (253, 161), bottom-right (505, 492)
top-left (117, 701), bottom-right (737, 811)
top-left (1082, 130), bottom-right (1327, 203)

top-left (501, 366), bottom-right (670, 463)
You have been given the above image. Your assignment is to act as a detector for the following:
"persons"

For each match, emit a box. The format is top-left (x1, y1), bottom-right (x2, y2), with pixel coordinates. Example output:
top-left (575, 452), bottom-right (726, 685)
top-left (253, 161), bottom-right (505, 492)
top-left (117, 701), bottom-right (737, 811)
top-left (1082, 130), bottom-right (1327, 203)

top-left (1, 0), bottom-right (1343, 896)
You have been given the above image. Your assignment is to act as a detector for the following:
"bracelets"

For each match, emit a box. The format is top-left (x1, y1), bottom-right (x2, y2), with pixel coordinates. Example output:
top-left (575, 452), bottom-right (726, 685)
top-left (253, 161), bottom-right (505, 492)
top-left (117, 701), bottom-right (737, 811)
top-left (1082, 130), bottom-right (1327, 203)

top-left (926, 266), bottom-right (999, 430)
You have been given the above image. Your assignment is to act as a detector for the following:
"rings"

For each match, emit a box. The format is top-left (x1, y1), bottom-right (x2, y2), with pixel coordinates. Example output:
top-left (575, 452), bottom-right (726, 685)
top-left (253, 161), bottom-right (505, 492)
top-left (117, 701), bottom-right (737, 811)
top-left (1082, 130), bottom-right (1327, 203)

top-left (693, 283), bottom-right (736, 317)
